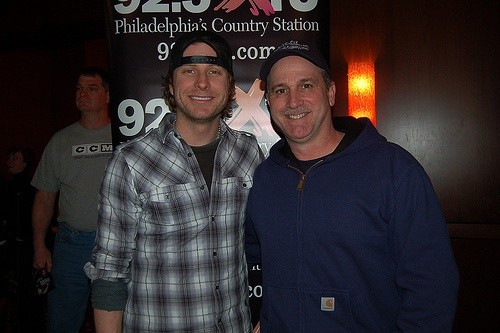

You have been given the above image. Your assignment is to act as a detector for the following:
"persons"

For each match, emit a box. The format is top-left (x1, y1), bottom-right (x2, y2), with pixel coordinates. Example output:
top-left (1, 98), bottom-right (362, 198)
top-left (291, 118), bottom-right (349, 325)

top-left (83, 30), bottom-right (266, 333)
top-left (243, 40), bottom-right (460, 333)
top-left (0, 145), bottom-right (56, 301)
top-left (30, 66), bottom-right (114, 333)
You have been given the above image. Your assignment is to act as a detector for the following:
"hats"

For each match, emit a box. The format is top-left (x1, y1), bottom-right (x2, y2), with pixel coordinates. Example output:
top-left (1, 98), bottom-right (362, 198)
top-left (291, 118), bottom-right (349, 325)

top-left (259, 39), bottom-right (331, 83)
top-left (168, 29), bottom-right (234, 74)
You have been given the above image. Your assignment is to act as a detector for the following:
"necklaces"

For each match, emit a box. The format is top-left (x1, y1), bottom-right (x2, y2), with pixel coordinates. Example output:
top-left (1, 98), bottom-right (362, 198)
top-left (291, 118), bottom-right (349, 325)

top-left (172, 116), bottom-right (221, 147)
